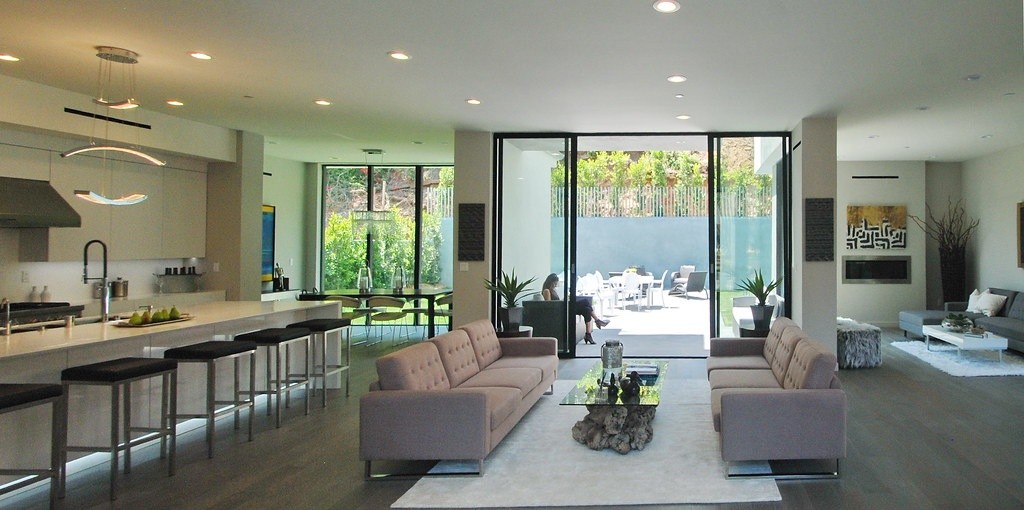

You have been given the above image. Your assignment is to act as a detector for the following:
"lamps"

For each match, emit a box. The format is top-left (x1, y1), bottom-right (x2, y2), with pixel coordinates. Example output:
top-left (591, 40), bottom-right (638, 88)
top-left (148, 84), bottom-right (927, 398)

top-left (391, 265), bottom-right (406, 295)
top-left (356, 259), bottom-right (374, 294)
top-left (60, 46), bottom-right (166, 206)
top-left (350, 148), bottom-right (395, 241)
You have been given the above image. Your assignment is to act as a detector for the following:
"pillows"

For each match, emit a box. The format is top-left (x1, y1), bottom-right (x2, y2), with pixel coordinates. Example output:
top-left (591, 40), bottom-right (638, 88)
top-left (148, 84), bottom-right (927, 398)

top-left (965, 288), bottom-right (1007, 317)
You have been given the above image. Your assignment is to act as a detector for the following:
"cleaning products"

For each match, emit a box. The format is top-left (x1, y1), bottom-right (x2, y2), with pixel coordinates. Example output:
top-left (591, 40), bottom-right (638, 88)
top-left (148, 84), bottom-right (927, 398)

top-left (29, 285), bottom-right (41, 303)
top-left (41, 285), bottom-right (52, 303)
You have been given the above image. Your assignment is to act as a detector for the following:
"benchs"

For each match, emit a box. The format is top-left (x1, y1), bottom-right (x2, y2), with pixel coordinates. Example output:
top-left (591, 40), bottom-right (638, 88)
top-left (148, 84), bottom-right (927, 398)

top-left (836, 317), bottom-right (883, 368)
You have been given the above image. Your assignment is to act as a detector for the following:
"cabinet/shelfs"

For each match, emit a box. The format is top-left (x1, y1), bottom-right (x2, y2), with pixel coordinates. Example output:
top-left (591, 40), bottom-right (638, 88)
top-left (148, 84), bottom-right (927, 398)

top-left (0, 144), bottom-right (208, 262)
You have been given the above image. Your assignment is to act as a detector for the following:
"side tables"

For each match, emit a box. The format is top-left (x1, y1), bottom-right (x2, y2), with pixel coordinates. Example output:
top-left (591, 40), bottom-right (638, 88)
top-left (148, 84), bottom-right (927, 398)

top-left (493, 326), bottom-right (533, 338)
top-left (739, 319), bottom-right (773, 338)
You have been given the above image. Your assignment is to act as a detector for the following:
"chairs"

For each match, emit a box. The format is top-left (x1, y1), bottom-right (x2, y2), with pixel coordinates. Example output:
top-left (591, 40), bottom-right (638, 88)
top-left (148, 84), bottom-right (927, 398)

top-left (575, 265), bottom-right (707, 320)
top-left (324, 292), bottom-right (454, 347)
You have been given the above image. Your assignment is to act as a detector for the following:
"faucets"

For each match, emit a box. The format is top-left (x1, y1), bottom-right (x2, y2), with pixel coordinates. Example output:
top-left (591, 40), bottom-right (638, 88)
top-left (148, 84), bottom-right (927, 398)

top-left (1, 296), bottom-right (11, 335)
top-left (82, 238), bottom-right (109, 323)
top-left (137, 304), bottom-right (153, 316)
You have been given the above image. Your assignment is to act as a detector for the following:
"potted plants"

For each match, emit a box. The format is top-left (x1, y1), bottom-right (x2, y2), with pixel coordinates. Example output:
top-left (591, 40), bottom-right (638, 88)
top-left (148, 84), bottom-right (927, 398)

top-left (734, 269), bottom-right (785, 321)
top-left (483, 267), bottom-right (539, 330)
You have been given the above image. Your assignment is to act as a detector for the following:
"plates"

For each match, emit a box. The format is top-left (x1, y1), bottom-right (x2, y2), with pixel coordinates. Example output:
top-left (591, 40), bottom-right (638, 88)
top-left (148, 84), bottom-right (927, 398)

top-left (109, 317), bottom-right (194, 327)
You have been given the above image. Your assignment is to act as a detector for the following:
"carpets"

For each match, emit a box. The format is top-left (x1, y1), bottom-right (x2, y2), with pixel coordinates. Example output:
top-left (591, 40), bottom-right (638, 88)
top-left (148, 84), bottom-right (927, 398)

top-left (890, 340), bottom-right (1024, 377)
top-left (390, 379), bottom-right (782, 508)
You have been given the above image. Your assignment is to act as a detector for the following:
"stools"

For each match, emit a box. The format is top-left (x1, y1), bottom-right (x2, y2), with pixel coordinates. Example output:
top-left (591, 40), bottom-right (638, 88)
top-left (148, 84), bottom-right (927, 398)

top-left (0, 317), bottom-right (351, 510)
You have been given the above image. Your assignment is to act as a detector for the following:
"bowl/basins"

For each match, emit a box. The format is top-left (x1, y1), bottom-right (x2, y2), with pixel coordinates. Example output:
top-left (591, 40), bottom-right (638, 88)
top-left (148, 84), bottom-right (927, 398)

top-left (157, 273), bottom-right (202, 292)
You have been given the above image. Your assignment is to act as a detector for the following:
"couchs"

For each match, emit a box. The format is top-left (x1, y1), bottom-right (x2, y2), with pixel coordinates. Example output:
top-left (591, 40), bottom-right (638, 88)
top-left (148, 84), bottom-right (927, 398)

top-left (732, 294), bottom-right (786, 338)
top-left (359, 319), bottom-right (559, 481)
top-left (522, 295), bottom-right (595, 353)
top-left (706, 317), bottom-right (848, 480)
top-left (898, 288), bottom-right (1024, 353)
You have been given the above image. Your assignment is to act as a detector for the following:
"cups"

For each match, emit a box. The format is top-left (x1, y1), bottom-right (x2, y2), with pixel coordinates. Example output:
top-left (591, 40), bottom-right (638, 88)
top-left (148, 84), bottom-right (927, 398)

top-left (108, 277), bottom-right (129, 298)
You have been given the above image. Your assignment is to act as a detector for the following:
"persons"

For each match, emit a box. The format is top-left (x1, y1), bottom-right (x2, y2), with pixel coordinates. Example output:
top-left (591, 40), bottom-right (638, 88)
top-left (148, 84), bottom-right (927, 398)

top-left (275, 262), bottom-right (285, 289)
top-left (541, 273), bottom-right (610, 344)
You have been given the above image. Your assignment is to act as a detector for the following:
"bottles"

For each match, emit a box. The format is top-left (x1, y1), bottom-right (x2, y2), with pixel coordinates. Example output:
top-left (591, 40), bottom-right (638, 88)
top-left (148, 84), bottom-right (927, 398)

top-left (40, 285), bottom-right (52, 302)
top-left (29, 286), bottom-right (41, 303)
top-left (601, 339), bottom-right (624, 369)
top-left (165, 265), bottom-right (197, 274)
top-left (357, 266), bottom-right (373, 294)
top-left (391, 265), bottom-right (407, 293)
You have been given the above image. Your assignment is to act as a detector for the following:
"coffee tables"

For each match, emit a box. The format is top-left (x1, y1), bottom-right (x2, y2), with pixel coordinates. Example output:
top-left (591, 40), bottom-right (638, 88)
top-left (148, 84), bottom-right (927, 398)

top-left (922, 325), bottom-right (1008, 363)
top-left (558, 360), bottom-right (668, 455)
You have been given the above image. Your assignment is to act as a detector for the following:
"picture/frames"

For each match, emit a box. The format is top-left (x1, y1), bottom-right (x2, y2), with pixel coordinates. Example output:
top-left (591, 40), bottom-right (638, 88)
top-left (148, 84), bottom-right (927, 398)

top-left (262, 204), bottom-right (276, 282)
top-left (1017, 202), bottom-right (1024, 268)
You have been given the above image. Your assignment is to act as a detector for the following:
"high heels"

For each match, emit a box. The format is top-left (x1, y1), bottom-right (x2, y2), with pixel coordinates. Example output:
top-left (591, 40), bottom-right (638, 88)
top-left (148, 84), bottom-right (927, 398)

top-left (583, 332), bottom-right (597, 344)
top-left (595, 319), bottom-right (610, 330)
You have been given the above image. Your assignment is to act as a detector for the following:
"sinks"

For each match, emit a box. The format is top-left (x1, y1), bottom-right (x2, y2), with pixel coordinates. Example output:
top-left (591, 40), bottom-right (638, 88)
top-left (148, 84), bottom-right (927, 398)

top-left (0, 311), bottom-right (135, 339)
top-left (0, 300), bottom-right (84, 316)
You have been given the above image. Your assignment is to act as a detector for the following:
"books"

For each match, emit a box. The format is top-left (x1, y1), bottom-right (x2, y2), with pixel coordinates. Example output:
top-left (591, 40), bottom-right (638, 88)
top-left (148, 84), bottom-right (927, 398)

top-left (625, 364), bottom-right (659, 375)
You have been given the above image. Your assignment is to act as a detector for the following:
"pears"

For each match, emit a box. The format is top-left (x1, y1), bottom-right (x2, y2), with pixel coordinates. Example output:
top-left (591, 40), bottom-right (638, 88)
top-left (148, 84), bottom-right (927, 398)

top-left (129, 305), bottom-right (180, 325)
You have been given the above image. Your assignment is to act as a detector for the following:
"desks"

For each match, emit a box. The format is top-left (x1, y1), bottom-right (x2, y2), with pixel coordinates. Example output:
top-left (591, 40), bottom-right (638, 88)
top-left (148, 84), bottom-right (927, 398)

top-left (606, 275), bottom-right (654, 314)
top-left (299, 290), bottom-right (454, 340)
top-left (0, 299), bottom-right (342, 486)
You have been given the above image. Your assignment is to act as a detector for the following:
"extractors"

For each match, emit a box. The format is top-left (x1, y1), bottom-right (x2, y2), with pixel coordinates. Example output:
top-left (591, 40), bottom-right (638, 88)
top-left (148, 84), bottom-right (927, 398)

top-left (0, 176), bottom-right (82, 229)
